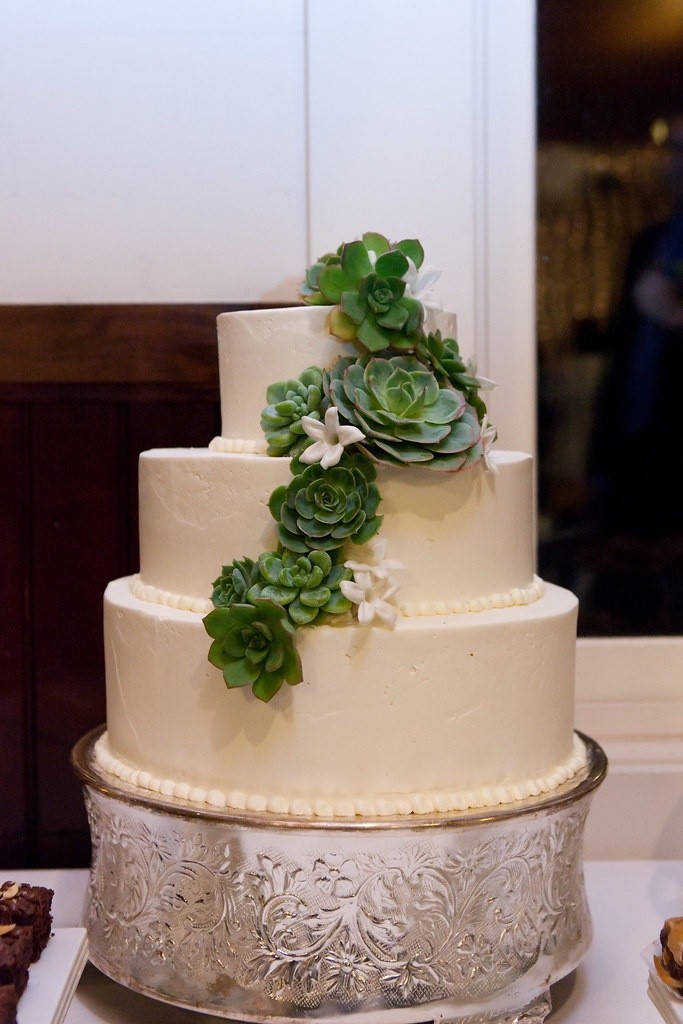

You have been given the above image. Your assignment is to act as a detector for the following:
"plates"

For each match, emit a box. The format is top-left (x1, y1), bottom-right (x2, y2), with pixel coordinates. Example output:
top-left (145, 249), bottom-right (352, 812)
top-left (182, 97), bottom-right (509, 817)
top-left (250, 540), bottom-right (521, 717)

top-left (639, 938), bottom-right (682, 1001)
top-left (14, 928), bottom-right (91, 1024)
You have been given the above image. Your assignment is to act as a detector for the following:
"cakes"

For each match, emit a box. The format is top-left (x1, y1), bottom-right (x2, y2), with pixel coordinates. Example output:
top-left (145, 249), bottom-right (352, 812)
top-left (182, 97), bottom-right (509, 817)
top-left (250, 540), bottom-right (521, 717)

top-left (0, 881), bottom-right (54, 1024)
top-left (95, 230), bottom-right (592, 821)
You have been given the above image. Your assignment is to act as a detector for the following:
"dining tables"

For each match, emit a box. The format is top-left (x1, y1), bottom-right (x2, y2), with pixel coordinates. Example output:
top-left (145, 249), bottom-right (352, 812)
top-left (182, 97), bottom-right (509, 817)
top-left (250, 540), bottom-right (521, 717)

top-left (0, 859), bottom-right (683, 1023)
